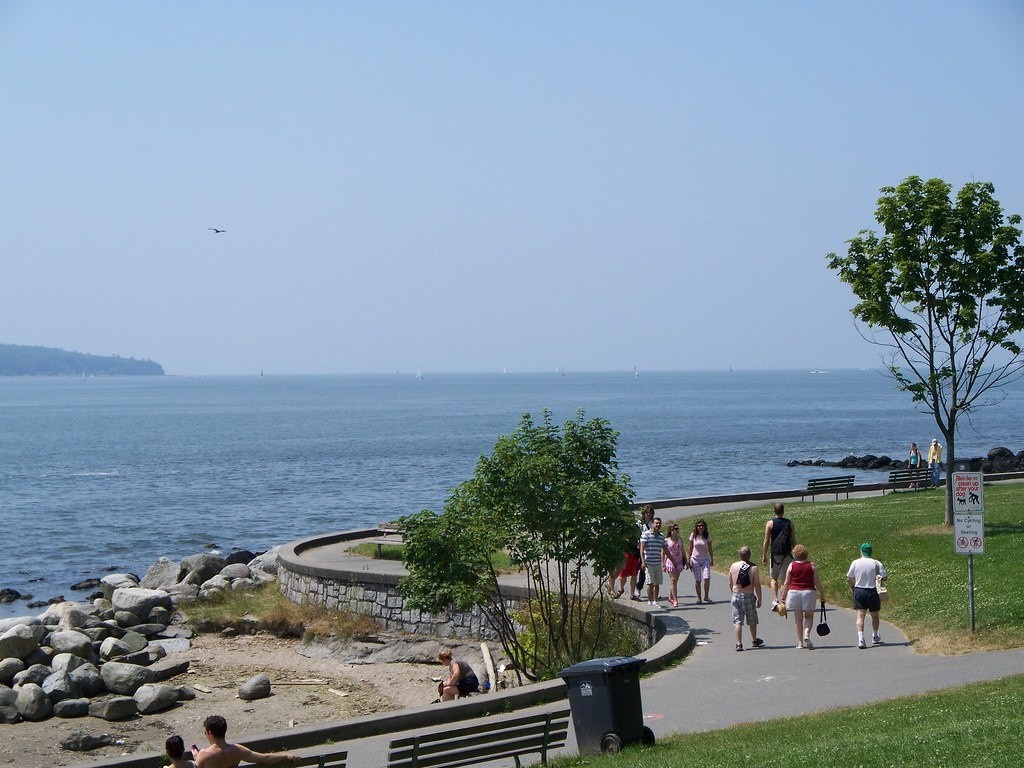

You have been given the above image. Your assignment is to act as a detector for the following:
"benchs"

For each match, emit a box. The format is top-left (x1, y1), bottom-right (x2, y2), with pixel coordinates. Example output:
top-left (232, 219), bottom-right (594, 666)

top-left (879, 467), bottom-right (934, 496)
top-left (370, 522), bottom-right (409, 558)
top-left (800, 475), bottom-right (855, 502)
top-left (238, 747), bottom-right (347, 768)
top-left (387, 707), bottom-right (571, 768)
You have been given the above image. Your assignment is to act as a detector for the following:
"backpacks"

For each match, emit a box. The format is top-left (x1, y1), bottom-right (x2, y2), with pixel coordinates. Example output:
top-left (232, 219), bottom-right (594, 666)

top-left (910, 449), bottom-right (921, 466)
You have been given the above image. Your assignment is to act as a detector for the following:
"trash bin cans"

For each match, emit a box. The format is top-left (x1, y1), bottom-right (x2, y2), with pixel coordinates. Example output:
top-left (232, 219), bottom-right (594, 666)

top-left (557, 655), bottom-right (657, 759)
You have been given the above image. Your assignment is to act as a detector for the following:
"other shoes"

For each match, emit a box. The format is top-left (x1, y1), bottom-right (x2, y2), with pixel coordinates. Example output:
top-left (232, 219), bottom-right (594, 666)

top-left (909, 483), bottom-right (919, 489)
top-left (612, 588), bottom-right (624, 599)
top-left (804, 635), bottom-right (813, 650)
top-left (697, 598), bottom-right (702, 604)
top-left (668, 597), bottom-right (678, 607)
top-left (630, 595), bottom-right (641, 602)
top-left (736, 643), bottom-right (743, 651)
top-left (704, 597), bottom-right (712, 601)
top-left (771, 600), bottom-right (779, 611)
top-left (752, 638), bottom-right (764, 647)
top-left (648, 601), bottom-right (662, 609)
top-left (796, 644), bottom-right (803, 648)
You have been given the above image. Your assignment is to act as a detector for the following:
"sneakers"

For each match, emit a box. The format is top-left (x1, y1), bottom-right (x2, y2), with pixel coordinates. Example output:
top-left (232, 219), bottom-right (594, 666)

top-left (871, 633), bottom-right (881, 644)
top-left (857, 640), bottom-right (866, 648)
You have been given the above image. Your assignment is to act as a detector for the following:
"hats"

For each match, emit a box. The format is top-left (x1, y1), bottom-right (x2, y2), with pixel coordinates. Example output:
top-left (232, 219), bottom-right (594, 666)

top-left (932, 439), bottom-right (937, 444)
top-left (777, 603), bottom-right (787, 619)
top-left (860, 543), bottom-right (872, 556)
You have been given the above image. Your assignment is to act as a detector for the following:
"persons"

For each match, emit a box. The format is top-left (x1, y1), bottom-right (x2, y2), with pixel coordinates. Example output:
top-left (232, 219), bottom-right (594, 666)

top-left (847, 542), bottom-right (887, 649)
top-left (439, 651), bottom-right (479, 702)
top-left (163, 735), bottom-right (196, 768)
top-left (661, 522), bottom-right (689, 607)
top-left (781, 544), bottom-right (827, 650)
top-left (640, 518), bottom-right (678, 606)
top-left (604, 505), bottom-right (661, 602)
top-left (928, 439), bottom-right (943, 488)
top-left (191, 715), bottom-right (301, 768)
top-left (687, 518), bottom-right (713, 605)
top-left (908, 442), bottom-right (920, 489)
top-left (762, 503), bottom-right (796, 612)
top-left (729, 546), bottom-right (764, 651)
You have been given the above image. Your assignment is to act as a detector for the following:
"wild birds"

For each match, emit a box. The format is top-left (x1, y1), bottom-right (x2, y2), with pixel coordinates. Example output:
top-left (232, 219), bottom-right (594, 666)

top-left (208, 226), bottom-right (226, 235)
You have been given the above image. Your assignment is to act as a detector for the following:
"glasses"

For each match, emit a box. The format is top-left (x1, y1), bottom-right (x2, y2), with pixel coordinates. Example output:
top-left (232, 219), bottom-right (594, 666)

top-left (696, 525), bottom-right (704, 527)
top-left (671, 528), bottom-right (679, 530)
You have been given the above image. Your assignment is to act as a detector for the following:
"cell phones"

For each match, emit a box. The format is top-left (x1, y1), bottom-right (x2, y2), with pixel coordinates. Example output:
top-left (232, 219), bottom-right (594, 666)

top-left (191, 744), bottom-right (199, 753)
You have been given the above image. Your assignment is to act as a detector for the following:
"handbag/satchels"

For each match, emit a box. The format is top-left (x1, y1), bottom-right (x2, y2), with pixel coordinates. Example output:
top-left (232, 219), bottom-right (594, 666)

top-left (771, 519), bottom-right (794, 568)
top-left (876, 574), bottom-right (888, 602)
top-left (817, 601), bottom-right (830, 636)
top-left (635, 561), bottom-right (646, 590)
